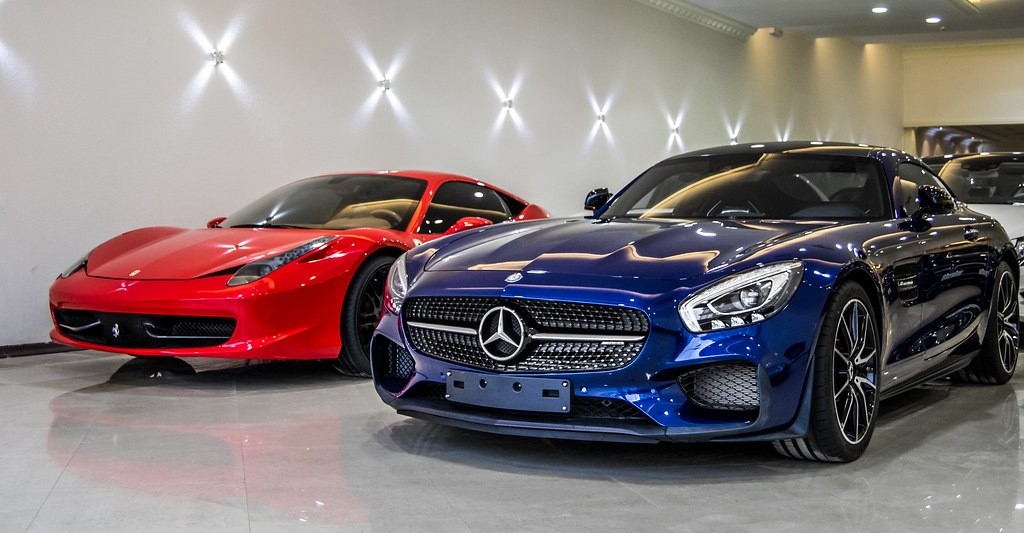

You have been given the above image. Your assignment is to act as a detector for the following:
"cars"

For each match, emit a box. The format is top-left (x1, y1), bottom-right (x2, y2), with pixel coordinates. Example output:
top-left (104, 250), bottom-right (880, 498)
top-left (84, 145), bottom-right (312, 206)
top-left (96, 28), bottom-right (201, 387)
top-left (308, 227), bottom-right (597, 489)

top-left (889, 151), bottom-right (1023, 324)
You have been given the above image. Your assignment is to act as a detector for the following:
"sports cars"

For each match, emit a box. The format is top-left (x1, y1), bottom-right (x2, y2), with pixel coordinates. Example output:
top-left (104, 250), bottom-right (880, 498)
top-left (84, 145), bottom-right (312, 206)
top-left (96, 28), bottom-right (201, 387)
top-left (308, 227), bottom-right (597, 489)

top-left (46, 168), bottom-right (553, 382)
top-left (368, 134), bottom-right (1021, 462)
